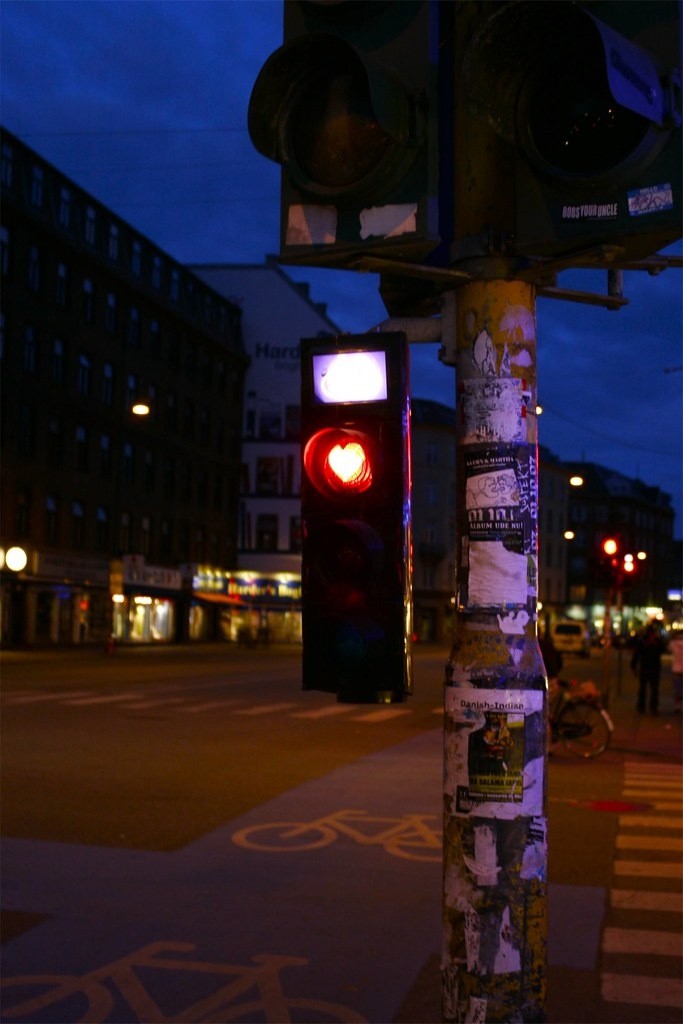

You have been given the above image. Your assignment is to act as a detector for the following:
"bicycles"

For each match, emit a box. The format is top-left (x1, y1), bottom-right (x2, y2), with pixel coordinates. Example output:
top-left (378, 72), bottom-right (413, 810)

top-left (543, 650), bottom-right (615, 763)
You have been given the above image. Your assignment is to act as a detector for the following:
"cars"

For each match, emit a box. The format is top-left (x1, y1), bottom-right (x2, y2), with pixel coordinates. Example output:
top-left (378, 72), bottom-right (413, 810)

top-left (553, 620), bottom-right (589, 657)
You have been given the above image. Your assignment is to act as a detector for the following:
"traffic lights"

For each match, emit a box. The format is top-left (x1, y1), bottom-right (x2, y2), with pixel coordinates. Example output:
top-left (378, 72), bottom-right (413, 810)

top-left (602, 536), bottom-right (620, 556)
top-left (295, 329), bottom-right (415, 700)
top-left (623, 561), bottom-right (638, 573)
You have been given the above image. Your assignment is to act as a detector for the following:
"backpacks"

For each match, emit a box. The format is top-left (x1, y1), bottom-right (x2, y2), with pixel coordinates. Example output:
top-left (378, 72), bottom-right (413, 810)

top-left (537, 639), bottom-right (563, 677)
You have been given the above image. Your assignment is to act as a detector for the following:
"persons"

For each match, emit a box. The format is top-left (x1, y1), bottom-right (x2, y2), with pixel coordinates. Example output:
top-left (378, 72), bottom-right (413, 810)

top-left (627, 617), bottom-right (683, 715)
top-left (536, 628), bottom-right (560, 761)
